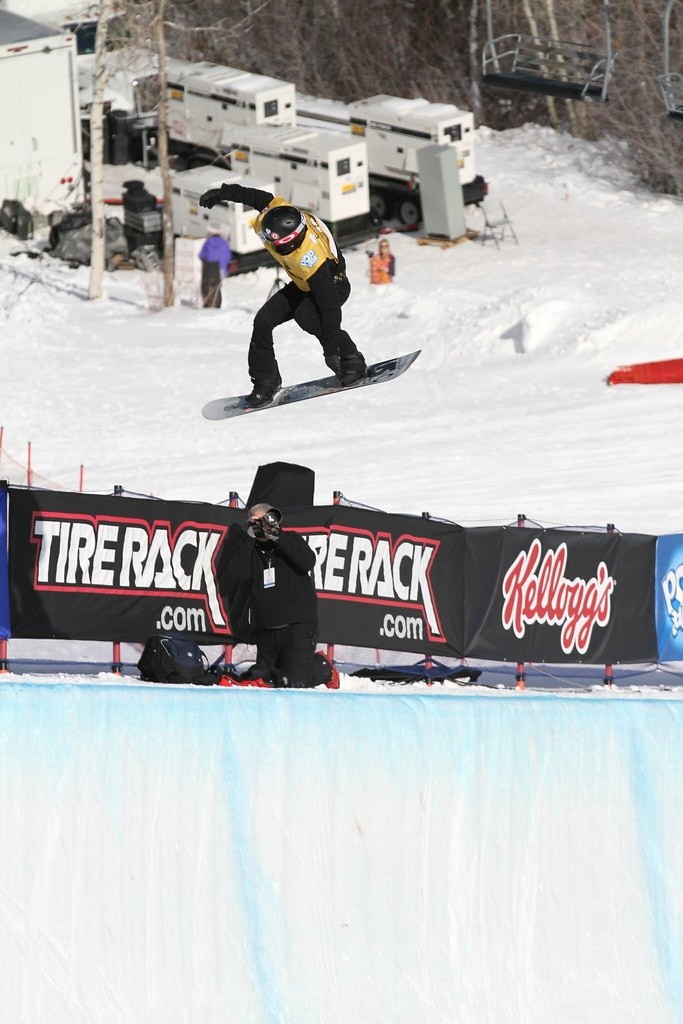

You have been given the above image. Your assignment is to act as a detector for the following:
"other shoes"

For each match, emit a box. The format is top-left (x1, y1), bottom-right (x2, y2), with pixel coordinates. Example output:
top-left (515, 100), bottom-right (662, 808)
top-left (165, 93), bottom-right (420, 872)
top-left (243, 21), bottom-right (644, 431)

top-left (316, 650), bottom-right (340, 689)
top-left (248, 379), bottom-right (282, 408)
top-left (340, 356), bottom-right (367, 386)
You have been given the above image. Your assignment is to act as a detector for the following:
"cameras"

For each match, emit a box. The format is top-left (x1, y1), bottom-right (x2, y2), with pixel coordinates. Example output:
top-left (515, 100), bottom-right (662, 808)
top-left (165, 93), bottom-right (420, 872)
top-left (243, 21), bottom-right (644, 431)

top-left (259, 508), bottom-right (282, 534)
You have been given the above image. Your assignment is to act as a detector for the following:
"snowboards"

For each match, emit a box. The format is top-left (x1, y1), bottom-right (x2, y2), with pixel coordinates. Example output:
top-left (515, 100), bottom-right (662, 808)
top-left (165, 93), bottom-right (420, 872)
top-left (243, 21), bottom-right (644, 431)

top-left (202, 349), bottom-right (422, 421)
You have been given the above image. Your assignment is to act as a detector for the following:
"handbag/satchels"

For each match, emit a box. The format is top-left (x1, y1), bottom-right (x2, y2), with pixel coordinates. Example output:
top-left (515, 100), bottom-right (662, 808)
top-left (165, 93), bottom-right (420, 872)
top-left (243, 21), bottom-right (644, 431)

top-left (137, 635), bottom-right (210, 684)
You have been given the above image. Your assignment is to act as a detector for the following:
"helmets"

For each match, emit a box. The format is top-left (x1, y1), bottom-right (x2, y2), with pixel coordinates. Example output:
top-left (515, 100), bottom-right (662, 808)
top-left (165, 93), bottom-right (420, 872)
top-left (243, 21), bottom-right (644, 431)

top-left (261, 205), bottom-right (306, 256)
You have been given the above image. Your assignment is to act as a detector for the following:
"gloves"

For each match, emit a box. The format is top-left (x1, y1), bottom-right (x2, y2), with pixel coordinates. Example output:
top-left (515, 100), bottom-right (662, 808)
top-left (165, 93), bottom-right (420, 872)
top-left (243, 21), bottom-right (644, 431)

top-left (199, 188), bottom-right (223, 210)
top-left (295, 298), bottom-right (316, 322)
top-left (264, 525), bottom-right (280, 542)
top-left (246, 525), bottom-right (261, 539)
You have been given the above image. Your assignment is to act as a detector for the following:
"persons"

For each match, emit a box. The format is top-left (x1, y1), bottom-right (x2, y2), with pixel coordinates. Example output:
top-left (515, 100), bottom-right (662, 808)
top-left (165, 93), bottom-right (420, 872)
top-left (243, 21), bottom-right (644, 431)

top-left (199, 183), bottom-right (367, 406)
top-left (370, 240), bottom-right (395, 284)
top-left (218, 503), bottom-right (340, 690)
top-left (199, 230), bottom-right (231, 287)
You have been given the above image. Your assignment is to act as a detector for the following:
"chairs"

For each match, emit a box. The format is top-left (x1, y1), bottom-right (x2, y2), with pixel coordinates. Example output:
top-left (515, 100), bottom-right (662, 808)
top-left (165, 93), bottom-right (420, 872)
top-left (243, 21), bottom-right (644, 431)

top-left (479, 198), bottom-right (522, 249)
